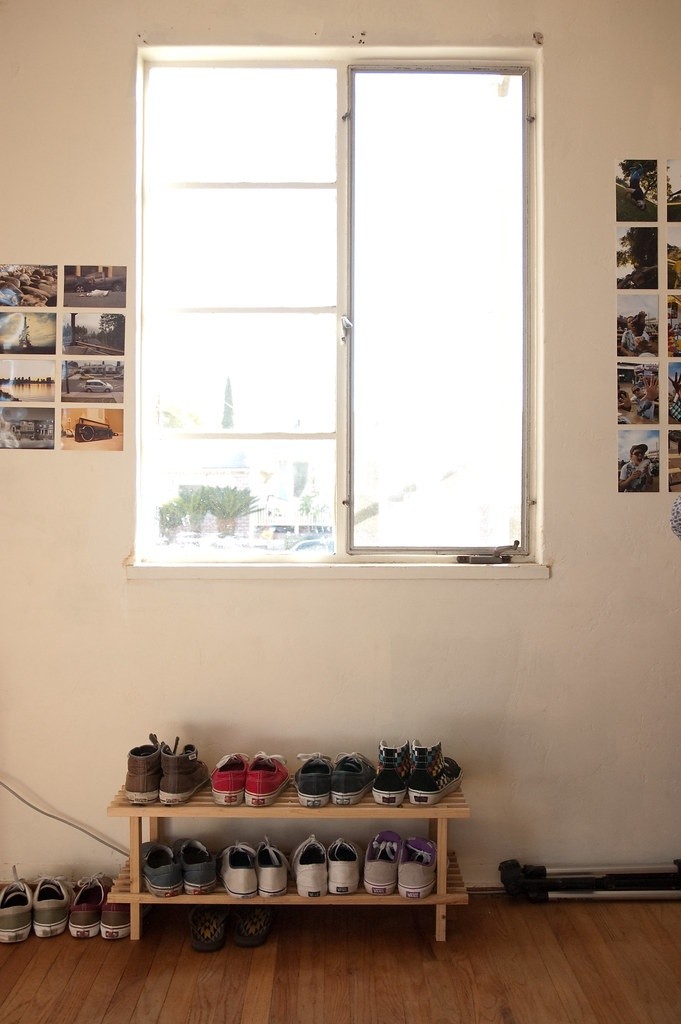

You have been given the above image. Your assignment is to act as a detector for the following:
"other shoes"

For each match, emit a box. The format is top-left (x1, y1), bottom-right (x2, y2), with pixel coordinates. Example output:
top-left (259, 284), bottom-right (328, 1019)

top-left (188, 906), bottom-right (227, 950)
top-left (235, 906), bottom-right (272, 948)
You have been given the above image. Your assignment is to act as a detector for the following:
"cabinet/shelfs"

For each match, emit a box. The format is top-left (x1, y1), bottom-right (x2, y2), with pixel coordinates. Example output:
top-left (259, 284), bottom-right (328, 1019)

top-left (106, 784), bottom-right (468, 941)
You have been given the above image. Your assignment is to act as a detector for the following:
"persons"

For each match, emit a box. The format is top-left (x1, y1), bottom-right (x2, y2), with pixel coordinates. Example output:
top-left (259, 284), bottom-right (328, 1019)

top-left (618, 374), bottom-right (659, 420)
top-left (672, 254), bottom-right (681, 283)
top-left (669, 322), bottom-right (681, 357)
top-left (668, 372), bottom-right (681, 422)
top-left (620, 444), bottom-right (653, 492)
top-left (617, 265), bottom-right (658, 289)
top-left (621, 316), bottom-right (649, 356)
top-left (624, 164), bottom-right (647, 211)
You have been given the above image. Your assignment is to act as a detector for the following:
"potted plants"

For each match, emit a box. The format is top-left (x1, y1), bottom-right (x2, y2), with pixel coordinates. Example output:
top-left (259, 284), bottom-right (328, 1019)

top-left (159, 486), bottom-right (264, 546)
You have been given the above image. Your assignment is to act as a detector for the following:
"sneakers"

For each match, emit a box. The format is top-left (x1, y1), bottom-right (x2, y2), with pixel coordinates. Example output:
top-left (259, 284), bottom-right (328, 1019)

top-left (99, 901), bottom-right (131, 940)
top-left (254, 834), bottom-right (288, 896)
top-left (399, 834), bottom-right (449, 899)
top-left (30, 874), bottom-right (77, 938)
top-left (327, 836), bottom-right (362, 895)
top-left (68, 872), bottom-right (113, 938)
top-left (373, 739), bottom-right (411, 806)
top-left (173, 835), bottom-right (218, 894)
top-left (361, 830), bottom-right (401, 894)
top-left (243, 750), bottom-right (291, 809)
top-left (289, 834), bottom-right (330, 898)
top-left (0, 865), bottom-right (33, 944)
top-left (139, 840), bottom-right (182, 896)
top-left (124, 733), bottom-right (166, 805)
top-left (330, 751), bottom-right (380, 805)
top-left (406, 742), bottom-right (463, 805)
top-left (217, 841), bottom-right (258, 899)
top-left (293, 751), bottom-right (331, 808)
top-left (209, 751), bottom-right (249, 806)
top-left (158, 737), bottom-right (209, 805)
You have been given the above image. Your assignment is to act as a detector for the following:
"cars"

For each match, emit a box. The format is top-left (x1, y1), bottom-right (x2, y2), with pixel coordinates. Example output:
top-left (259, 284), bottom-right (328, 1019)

top-left (64, 272), bottom-right (126, 292)
top-left (84, 379), bottom-right (114, 393)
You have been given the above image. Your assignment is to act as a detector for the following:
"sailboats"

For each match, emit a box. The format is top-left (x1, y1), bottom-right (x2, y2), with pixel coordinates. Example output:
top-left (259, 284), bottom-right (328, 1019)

top-left (18, 316), bottom-right (34, 352)
